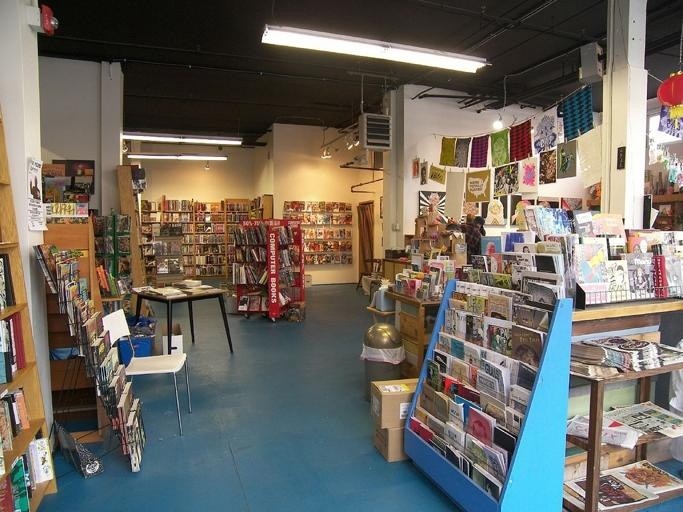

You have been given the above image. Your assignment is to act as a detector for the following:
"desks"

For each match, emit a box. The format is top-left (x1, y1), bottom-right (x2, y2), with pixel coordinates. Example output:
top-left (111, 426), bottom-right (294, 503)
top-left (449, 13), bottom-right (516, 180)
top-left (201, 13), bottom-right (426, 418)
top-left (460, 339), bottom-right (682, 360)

top-left (134, 283), bottom-right (234, 355)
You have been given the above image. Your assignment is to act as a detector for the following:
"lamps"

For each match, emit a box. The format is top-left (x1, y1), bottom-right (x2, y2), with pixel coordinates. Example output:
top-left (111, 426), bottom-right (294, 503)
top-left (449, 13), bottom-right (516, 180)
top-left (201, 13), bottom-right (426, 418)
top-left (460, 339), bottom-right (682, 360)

top-left (126, 151), bottom-right (229, 164)
top-left (317, 124), bottom-right (360, 159)
top-left (204, 159), bottom-right (213, 172)
top-left (260, 5), bottom-right (493, 73)
top-left (121, 130), bottom-right (243, 149)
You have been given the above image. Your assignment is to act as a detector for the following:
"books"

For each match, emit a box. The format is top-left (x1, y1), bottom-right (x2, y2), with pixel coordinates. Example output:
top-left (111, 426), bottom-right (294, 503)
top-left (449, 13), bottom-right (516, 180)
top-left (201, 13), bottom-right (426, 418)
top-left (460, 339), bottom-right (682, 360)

top-left (0, 190), bottom-right (148, 511)
top-left (147, 195), bottom-right (353, 313)
top-left (393, 205), bottom-right (683, 512)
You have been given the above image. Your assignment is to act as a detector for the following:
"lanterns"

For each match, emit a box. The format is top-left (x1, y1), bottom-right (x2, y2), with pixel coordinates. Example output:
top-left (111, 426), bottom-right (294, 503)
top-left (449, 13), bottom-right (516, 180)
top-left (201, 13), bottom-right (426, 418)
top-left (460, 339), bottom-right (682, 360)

top-left (656, 70), bottom-right (683, 120)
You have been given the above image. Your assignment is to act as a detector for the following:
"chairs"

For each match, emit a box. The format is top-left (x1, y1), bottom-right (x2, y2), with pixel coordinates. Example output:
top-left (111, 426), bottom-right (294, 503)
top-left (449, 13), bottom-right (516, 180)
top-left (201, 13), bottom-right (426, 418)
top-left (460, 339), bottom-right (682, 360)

top-left (100, 309), bottom-right (192, 438)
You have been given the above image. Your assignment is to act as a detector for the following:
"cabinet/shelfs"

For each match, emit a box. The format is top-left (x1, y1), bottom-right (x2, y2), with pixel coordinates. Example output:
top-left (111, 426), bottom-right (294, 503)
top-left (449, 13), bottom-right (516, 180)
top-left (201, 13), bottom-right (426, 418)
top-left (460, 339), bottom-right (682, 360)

top-left (231, 218), bottom-right (307, 319)
top-left (138, 193), bottom-right (272, 282)
top-left (281, 201), bottom-right (353, 264)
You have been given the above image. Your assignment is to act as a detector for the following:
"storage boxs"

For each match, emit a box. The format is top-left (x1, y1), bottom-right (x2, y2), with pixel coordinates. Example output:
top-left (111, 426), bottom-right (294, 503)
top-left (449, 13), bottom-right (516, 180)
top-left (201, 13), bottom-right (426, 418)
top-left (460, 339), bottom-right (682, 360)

top-left (160, 322), bottom-right (184, 356)
